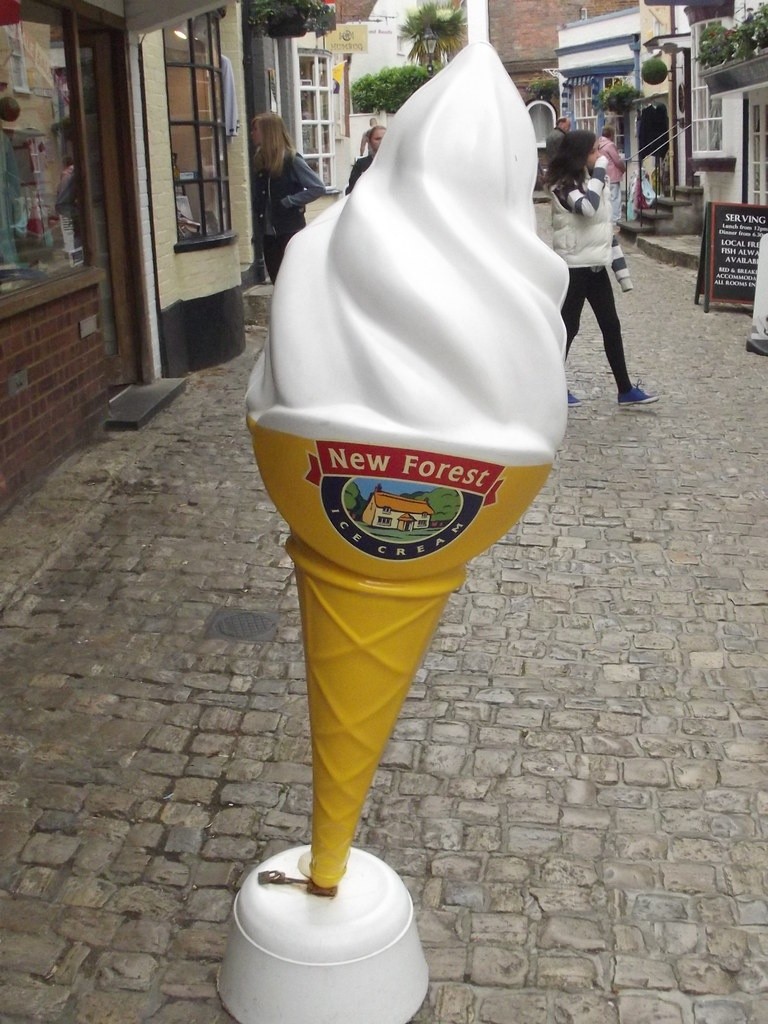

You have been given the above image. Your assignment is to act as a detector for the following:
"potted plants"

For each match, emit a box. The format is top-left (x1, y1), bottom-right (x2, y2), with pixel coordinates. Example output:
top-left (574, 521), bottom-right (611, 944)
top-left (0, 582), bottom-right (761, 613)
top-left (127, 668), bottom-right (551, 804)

top-left (591, 79), bottom-right (645, 112)
top-left (246, 0), bottom-right (337, 40)
top-left (528, 75), bottom-right (560, 101)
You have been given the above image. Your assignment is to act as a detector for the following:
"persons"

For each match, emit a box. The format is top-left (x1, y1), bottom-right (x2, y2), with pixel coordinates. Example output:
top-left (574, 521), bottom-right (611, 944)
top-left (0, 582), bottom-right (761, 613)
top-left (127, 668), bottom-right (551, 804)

top-left (250, 112), bottom-right (324, 286)
top-left (542, 129), bottom-right (658, 407)
top-left (545, 117), bottom-right (571, 162)
top-left (55, 157), bottom-right (74, 181)
top-left (343, 126), bottom-right (387, 196)
top-left (598, 126), bottom-right (627, 226)
top-left (359, 117), bottom-right (378, 157)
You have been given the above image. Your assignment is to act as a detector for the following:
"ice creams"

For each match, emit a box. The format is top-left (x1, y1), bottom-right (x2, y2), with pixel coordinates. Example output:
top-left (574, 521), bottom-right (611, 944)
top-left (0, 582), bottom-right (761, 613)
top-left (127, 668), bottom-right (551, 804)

top-left (245, 35), bottom-right (569, 897)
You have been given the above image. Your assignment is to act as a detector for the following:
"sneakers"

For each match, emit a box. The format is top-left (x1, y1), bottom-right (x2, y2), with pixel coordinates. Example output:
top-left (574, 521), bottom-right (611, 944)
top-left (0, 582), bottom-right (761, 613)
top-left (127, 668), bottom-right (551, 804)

top-left (568, 393), bottom-right (582, 407)
top-left (618, 384), bottom-right (659, 406)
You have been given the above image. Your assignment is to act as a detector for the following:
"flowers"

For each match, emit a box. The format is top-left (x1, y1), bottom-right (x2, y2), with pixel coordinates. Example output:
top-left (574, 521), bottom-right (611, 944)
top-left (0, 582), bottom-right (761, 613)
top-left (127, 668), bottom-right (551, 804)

top-left (693, 2), bottom-right (768, 69)
